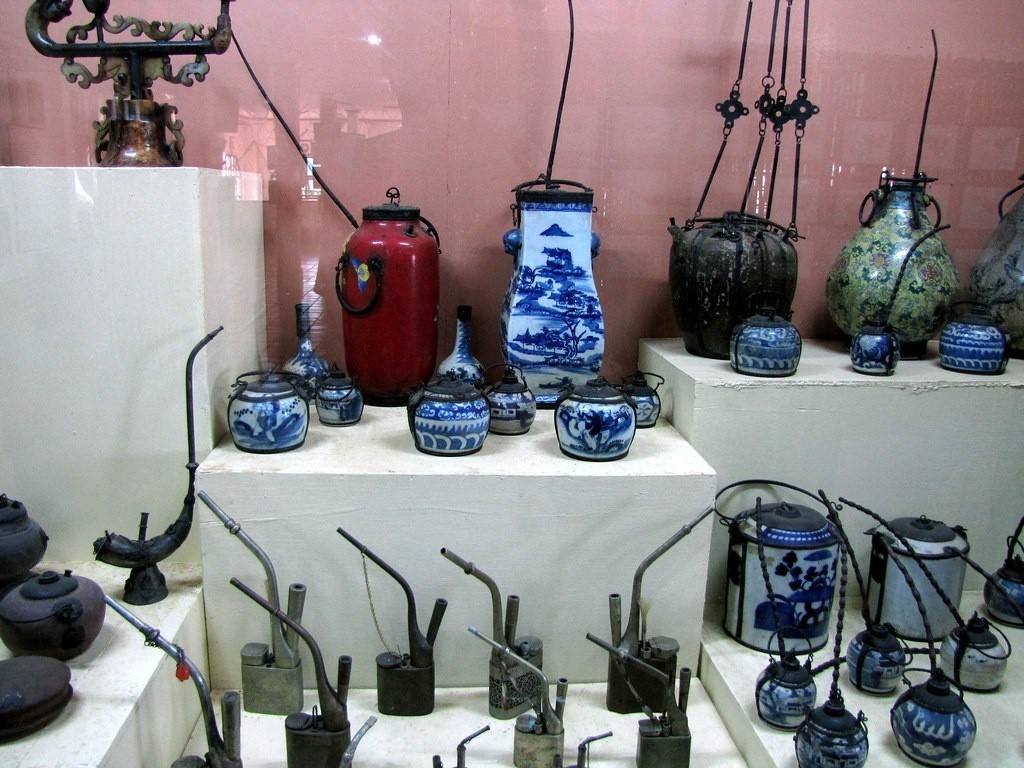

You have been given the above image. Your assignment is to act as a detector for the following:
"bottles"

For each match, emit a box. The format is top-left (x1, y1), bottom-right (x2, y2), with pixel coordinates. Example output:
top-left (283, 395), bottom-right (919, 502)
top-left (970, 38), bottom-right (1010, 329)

top-left (435, 306), bottom-right (487, 392)
top-left (282, 304), bottom-right (331, 405)
